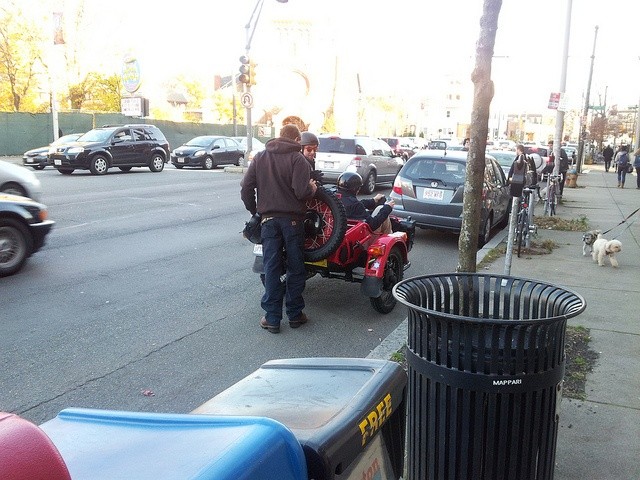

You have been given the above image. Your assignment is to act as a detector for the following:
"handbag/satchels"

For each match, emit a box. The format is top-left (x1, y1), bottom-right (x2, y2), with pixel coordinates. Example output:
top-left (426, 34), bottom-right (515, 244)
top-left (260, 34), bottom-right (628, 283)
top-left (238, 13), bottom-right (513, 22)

top-left (626, 163), bottom-right (633, 173)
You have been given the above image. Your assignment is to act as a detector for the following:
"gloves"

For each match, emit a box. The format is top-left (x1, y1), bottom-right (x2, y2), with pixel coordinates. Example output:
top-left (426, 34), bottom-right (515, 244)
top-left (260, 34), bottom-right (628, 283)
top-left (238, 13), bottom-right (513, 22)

top-left (310, 170), bottom-right (324, 181)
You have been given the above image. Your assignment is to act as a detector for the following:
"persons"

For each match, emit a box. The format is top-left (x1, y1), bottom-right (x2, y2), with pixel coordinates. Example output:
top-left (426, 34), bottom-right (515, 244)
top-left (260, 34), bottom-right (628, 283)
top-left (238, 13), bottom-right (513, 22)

top-left (633, 145), bottom-right (640, 189)
top-left (558, 147), bottom-right (569, 205)
top-left (603, 144), bottom-right (614, 172)
top-left (462, 138), bottom-right (470, 148)
top-left (297, 129), bottom-right (320, 170)
top-left (327, 170), bottom-right (396, 235)
top-left (506, 144), bottom-right (526, 197)
top-left (614, 145), bottom-right (631, 188)
top-left (547, 140), bottom-right (554, 150)
top-left (238, 124), bottom-right (318, 333)
top-left (614, 146), bottom-right (621, 173)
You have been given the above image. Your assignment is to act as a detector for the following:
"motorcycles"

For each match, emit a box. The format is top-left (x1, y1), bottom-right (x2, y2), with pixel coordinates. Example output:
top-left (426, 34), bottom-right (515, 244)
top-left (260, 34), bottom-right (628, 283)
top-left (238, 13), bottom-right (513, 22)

top-left (252, 186), bottom-right (416, 314)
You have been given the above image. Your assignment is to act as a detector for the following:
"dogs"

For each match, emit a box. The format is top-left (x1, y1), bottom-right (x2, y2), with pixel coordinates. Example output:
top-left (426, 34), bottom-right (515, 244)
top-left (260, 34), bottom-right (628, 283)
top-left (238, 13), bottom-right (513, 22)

top-left (591, 233), bottom-right (623, 267)
top-left (582, 229), bottom-right (603, 256)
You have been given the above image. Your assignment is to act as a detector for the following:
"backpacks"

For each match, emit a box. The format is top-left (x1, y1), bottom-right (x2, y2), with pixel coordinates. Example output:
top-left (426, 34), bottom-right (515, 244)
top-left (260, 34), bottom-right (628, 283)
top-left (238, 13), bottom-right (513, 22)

top-left (618, 153), bottom-right (629, 171)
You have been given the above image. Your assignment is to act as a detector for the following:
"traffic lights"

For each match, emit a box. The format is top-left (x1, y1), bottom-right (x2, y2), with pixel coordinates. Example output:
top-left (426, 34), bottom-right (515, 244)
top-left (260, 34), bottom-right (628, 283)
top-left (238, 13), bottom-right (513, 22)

top-left (239, 55), bottom-right (250, 84)
top-left (250, 60), bottom-right (259, 88)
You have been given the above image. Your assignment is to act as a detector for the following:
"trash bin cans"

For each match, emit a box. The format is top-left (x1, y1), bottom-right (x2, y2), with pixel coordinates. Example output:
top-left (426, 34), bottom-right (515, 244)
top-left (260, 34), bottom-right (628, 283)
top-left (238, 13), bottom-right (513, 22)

top-left (36, 408), bottom-right (306, 476)
top-left (390, 271), bottom-right (585, 475)
top-left (188, 359), bottom-right (409, 475)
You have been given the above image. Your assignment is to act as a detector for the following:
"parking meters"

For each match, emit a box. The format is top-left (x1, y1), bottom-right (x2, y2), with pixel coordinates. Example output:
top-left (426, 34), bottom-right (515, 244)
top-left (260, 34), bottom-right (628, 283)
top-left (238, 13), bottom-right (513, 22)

top-left (500, 154), bottom-right (528, 286)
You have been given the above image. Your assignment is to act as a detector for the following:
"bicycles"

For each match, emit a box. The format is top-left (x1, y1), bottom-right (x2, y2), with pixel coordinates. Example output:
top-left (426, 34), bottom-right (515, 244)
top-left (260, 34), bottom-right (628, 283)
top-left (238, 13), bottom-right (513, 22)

top-left (513, 184), bottom-right (542, 257)
top-left (540, 173), bottom-right (564, 217)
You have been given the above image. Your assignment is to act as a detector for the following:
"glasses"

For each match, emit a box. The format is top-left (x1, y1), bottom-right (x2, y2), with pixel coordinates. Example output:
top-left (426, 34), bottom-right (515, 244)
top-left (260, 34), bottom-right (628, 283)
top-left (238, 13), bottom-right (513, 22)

top-left (304, 146), bottom-right (319, 152)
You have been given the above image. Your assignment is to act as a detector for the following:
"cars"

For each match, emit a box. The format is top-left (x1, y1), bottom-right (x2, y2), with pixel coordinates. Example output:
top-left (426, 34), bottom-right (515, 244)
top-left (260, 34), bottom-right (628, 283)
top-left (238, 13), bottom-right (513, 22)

top-left (561, 147), bottom-right (578, 164)
top-left (486, 140), bottom-right (517, 148)
top-left (385, 148), bottom-right (512, 247)
top-left (22, 132), bottom-right (85, 169)
top-left (171, 135), bottom-right (246, 169)
top-left (231, 136), bottom-right (266, 152)
top-left (413, 137), bottom-right (464, 148)
top-left (0, 191), bottom-right (56, 277)
top-left (0, 158), bottom-right (44, 206)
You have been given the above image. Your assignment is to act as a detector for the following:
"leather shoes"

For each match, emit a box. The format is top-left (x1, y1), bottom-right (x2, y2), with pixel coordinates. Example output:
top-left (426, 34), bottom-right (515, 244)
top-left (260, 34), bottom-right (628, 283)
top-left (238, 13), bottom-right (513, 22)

top-left (288, 313), bottom-right (307, 328)
top-left (259, 316), bottom-right (280, 333)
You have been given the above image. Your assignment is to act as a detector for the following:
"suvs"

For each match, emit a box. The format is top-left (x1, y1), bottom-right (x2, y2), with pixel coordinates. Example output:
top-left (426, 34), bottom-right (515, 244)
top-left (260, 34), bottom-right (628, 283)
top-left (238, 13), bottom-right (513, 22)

top-left (48, 124), bottom-right (171, 176)
top-left (522, 145), bottom-right (555, 181)
top-left (314, 134), bottom-right (405, 195)
top-left (384, 138), bottom-right (417, 159)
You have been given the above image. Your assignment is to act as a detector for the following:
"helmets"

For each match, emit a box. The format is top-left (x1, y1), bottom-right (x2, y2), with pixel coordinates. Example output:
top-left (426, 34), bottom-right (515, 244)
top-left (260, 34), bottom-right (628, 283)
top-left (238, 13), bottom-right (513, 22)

top-left (243, 211), bottom-right (263, 244)
top-left (298, 132), bottom-right (318, 154)
top-left (338, 172), bottom-right (364, 198)
